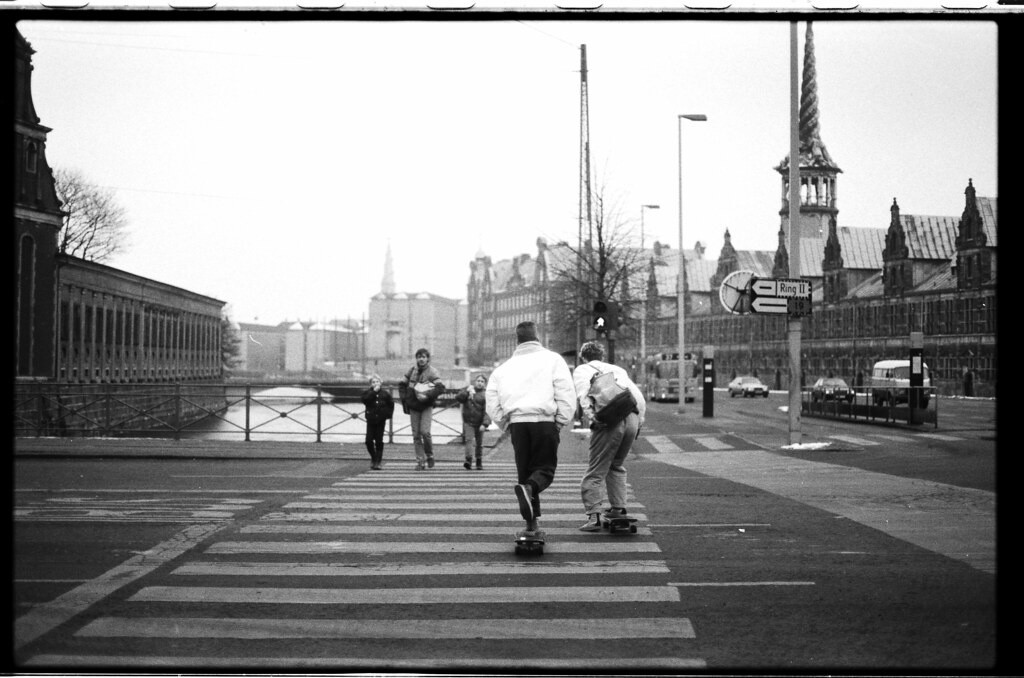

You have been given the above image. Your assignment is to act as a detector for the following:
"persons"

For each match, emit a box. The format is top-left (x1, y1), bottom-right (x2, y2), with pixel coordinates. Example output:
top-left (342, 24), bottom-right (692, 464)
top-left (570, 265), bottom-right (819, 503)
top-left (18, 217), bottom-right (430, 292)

top-left (361, 373), bottom-right (394, 470)
top-left (753, 368), bottom-right (757, 377)
top-left (456, 375), bottom-right (487, 470)
top-left (485, 322), bottom-right (577, 536)
top-left (856, 370), bottom-right (863, 393)
top-left (400, 349), bottom-right (446, 471)
top-left (692, 362), bottom-right (698, 378)
top-left (573, 342), bottom-right (646, 531)
top-left (962, 365), bottom-right (973, 397)
top-left (729, 368), bottom-right (736, 383)
top-left (800, 368), bottom-right (806, 387)
top-left (774, 369), bottom-right (781, 390)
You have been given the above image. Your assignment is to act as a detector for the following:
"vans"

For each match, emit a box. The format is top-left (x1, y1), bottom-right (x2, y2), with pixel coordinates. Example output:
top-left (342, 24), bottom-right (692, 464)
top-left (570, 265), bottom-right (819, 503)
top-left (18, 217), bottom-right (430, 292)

top-left (871, 359), bottom-right (931, 409)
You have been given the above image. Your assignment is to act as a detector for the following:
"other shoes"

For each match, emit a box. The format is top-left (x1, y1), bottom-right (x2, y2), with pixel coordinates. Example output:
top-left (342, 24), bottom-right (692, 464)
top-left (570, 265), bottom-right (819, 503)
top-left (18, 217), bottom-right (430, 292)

top-left (416, 464), bottom-right (425, 470)
top-left (476, 460), bottom-right (483, 469)
top-left (428, 453), bottom-right (434, 469)
top-left (514, 484), bottom-right (534, 521)
top-left (579, 514), bottom-right (602, 532)
top-left (369, 455), bottom-right (381, 470)
top-left (603, 505), bottom-right (627, 519)
top-left (526, 520), bottom-right (541, 536)
top-left (464, 461), bottom-right (471, 470)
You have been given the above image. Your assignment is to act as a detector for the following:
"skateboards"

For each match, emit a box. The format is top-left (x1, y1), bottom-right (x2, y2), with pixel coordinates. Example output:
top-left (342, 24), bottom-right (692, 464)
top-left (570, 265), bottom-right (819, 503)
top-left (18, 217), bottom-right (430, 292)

top-left (601, 514), bottom-right (638, 533)
top-left (514, 529), bottom-right (546, 555)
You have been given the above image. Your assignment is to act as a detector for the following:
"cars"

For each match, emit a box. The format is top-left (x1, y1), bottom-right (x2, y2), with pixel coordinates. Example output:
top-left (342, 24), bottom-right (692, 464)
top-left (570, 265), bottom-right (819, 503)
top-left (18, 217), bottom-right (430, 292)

top-left (727, 375), bottom-right (769, 399)
top-left (811, 378), bottom-right (855, 404)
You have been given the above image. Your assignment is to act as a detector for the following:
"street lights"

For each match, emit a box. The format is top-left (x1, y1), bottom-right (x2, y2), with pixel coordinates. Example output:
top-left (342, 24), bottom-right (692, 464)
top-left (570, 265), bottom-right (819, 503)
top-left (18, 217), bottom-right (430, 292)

top-left (678, 114), bottom-right (707, 413)
top-left (640, 203), bottom-right (660, 402)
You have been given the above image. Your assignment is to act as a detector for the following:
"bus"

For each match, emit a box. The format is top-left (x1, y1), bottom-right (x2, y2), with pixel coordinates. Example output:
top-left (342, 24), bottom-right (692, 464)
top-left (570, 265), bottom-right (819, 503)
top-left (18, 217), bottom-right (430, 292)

top-left (645, 351), bottom-right (701, 404)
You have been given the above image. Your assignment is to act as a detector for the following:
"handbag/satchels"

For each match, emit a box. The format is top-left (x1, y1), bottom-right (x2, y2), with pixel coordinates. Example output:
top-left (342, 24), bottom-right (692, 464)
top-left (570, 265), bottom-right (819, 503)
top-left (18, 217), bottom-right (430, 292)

top-left (596, 389), bottom-right (638, 427)
top-left (588, 366), bottom-right (627, 414)
top-left (402, 399), bottom-right (410, 414)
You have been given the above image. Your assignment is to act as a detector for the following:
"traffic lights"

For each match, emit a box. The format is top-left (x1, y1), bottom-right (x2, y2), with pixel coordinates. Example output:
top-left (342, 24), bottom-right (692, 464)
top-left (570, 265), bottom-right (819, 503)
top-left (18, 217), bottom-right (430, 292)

top-left (593, 300), bottom-right (619, 331)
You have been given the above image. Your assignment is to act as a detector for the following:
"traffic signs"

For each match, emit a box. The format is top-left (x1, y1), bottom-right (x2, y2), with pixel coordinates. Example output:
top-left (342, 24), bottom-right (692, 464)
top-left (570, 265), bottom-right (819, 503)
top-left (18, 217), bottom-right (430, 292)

top-left (749, 277), bottom-right (813, 319)
